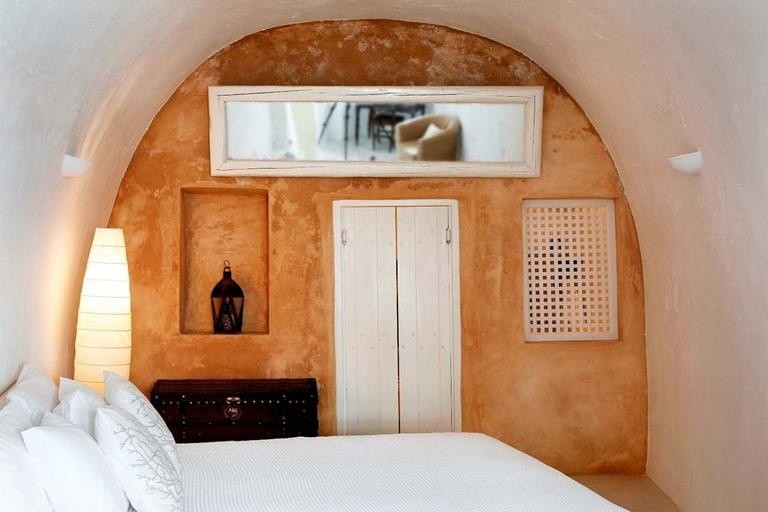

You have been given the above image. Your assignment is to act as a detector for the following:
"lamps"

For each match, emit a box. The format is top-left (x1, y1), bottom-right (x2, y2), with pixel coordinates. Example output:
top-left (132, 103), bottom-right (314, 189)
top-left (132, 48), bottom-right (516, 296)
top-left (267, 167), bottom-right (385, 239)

top-left (71, 227), bottom-right (133, 398)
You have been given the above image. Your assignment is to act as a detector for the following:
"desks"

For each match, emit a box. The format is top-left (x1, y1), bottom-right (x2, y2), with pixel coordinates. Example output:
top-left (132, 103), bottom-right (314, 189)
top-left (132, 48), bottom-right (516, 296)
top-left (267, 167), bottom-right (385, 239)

top-left (354, 103), bottom-right (425, 146)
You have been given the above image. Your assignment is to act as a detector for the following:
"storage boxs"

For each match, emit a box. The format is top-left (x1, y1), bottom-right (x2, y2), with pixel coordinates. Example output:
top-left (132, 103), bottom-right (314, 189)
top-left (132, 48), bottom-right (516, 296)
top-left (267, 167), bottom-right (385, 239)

top-left (151, 378), bottom-right (320, 444)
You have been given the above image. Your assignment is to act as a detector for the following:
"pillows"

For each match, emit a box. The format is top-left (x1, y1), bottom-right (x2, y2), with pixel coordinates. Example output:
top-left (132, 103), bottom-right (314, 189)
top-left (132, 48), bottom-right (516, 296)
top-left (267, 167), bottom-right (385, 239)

top-left (423, 122), bottom-right (442, 139)
top-left (0, 359), bottom-right (189, 511)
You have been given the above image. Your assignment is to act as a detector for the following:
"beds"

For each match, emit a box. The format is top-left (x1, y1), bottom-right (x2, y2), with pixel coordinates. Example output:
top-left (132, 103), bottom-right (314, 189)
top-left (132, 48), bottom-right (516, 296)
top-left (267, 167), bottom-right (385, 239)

top-left (0, 376), bottom-right (631, 511)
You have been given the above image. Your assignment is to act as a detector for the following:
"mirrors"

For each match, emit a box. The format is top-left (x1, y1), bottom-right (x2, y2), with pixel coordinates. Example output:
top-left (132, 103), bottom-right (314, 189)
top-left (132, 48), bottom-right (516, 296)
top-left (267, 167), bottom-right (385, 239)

top-left (206, 85), bottom-right (544, 179)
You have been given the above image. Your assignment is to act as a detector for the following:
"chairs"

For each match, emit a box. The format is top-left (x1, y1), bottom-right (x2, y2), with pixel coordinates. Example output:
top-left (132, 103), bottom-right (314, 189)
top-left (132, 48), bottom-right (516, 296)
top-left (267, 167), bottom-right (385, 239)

top-left (394, 115), bottom-right (460, 163)
top-left (369, 103), bottom-right (405, 154)
